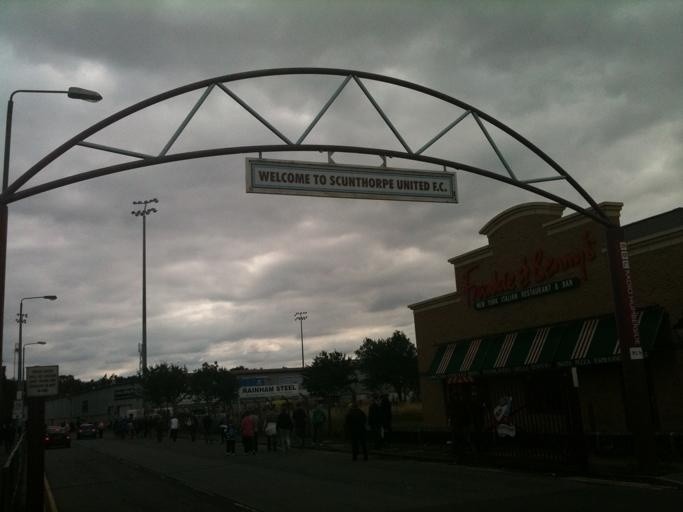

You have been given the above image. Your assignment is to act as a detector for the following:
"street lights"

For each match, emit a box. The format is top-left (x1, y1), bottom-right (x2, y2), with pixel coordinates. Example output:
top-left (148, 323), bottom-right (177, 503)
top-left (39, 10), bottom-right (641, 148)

top-left (132, 195), bottom-right (162, 380)
top-left (292, 308), bottom-right (314, 375)
top-left (0, 87), bottom-right (102, 430)
top-left (11, 291), bottom-right (59, 406)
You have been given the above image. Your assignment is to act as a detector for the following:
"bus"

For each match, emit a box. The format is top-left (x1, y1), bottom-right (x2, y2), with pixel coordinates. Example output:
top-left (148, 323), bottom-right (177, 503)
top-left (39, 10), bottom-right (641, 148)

top-left (125, 407), bottom-right (174, 421)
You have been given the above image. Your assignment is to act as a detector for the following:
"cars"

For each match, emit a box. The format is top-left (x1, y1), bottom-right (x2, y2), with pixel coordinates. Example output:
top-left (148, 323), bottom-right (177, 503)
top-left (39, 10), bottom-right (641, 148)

top-left (76, 423), bottom-right (98, 441)
top-left (43, 423), bottom-right (71, 449)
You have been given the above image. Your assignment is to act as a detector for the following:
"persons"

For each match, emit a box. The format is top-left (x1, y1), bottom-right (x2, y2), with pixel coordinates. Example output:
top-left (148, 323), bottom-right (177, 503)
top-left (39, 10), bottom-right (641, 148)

top-left (367, 398), bottom-right (382, 447)
top-left (96, 401), bottom-right (327, 457)
top-left (344, 401), bottom-right (368, 462)
top-left (380, 393), bottom-right (392, 443)
